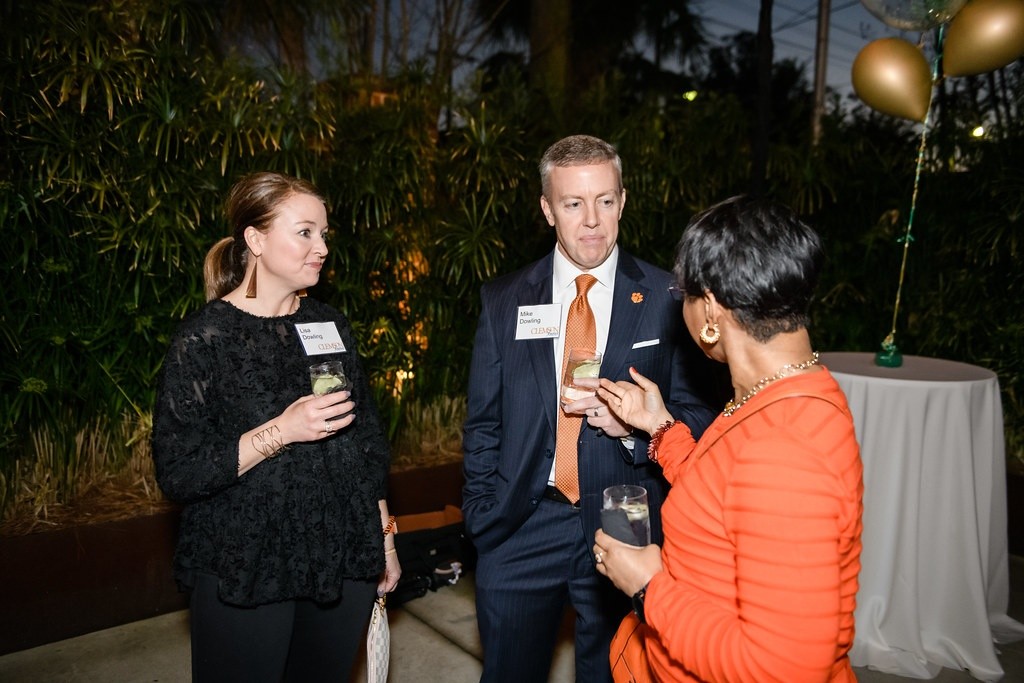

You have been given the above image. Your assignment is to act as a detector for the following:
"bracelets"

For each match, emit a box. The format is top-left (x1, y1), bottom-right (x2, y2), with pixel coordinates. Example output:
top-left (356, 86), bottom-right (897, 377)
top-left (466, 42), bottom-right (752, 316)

top-left (384, 549), bottom-right (396, 555)
top-left (647, 419), bottom-right (682, 464)
top-left (251, 424), bottom-right (291, 461)
top-left (383, 515), bottom-right (398, 542)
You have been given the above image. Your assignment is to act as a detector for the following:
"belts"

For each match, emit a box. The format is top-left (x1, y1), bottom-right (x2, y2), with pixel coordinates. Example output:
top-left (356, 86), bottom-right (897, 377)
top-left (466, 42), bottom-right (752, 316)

top-left (541, 484), bottom-right (581, 507)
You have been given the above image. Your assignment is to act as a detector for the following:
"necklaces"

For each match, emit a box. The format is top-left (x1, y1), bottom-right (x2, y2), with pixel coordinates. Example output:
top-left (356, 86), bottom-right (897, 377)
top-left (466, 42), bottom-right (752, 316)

top-left (724, 350), bottom-right (819, 418)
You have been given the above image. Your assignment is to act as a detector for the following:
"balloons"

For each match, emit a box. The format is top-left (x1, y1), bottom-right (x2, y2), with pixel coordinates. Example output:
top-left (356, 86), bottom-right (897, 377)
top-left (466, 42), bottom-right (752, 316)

top-left (852, 37), bottom-right (931, 122)
top-left (943, 0), bottom-right (1024, 78)
top-left (860, 0), bottom-right (968, 31)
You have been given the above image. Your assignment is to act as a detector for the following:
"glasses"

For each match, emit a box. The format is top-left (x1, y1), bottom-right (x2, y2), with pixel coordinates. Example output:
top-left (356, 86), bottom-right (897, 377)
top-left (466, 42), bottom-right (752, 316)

top-left (667, 279), bottom-right (693, 300)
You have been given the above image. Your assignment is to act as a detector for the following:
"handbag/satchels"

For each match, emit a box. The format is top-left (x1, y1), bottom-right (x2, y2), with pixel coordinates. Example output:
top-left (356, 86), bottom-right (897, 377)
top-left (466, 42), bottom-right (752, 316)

top-left (367, 515), bottom-right (395, 683)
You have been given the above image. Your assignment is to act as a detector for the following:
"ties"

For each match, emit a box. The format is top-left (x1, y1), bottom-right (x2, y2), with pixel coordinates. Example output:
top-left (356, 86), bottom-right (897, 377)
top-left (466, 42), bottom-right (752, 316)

top-left (554, 274), bottom-right (599, 504)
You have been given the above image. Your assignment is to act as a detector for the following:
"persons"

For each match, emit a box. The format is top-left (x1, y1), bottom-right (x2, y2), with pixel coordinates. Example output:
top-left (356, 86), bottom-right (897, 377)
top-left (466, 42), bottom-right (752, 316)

top-left (591, 192), bottom-right (865, 683)
top-left (147, 170), bottom-right (402, 683)
top-left (462, 134), bottom-right (728, 683)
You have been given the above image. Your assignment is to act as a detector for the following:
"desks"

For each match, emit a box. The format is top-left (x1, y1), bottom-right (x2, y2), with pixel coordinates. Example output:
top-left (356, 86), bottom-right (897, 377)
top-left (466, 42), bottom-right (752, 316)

top-left (814, 353), bottom-right (1023, 683)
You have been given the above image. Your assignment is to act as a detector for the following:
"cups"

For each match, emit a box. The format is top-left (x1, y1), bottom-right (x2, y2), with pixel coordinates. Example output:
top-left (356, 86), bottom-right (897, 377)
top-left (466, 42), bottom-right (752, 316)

top-left (309, 361), bottom-right (357, 421)
top-left (560, 346), bottom-right (602, 414)
top-left (603, 485), bottom-right (651, 546)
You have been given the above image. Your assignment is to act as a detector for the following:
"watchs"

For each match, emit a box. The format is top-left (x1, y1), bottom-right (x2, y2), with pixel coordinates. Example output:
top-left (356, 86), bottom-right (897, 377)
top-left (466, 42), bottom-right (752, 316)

top-left (632, 580), bottom-right (650, 623)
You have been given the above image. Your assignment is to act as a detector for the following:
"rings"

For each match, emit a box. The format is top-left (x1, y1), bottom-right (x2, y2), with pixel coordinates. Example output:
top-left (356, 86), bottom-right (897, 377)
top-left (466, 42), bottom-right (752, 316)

top-left (594, 408), bottom-right (598, 417)
top-left (326, 421), bottom-right (332, 433)
top-left (595, 549), bottom-right (605, 563)
